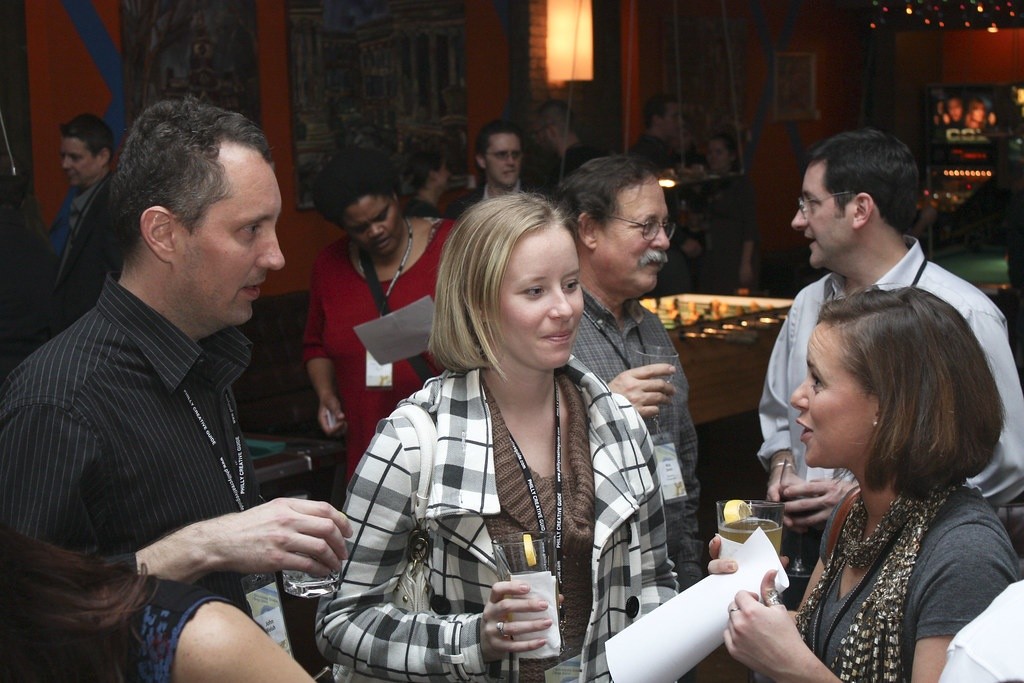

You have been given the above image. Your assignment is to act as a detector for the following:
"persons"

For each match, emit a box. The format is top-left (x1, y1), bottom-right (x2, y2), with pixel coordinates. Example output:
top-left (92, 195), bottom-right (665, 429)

top-left (402, 93), bottom-right (759, 295)
top-left (0, 92), bottom-right (354, 683)
top-left (707, 127), bottom-right (1024, 683)
top-left (302, 154), bottom-right (704, 683)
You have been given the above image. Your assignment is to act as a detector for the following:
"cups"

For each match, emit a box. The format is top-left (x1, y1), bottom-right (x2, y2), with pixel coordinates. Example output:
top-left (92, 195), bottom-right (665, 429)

top-left (717, 499), bottom-right (785, 558)
top-left (283, 551), bottom-right (342, 598)
top-left (492, 531), bottom-right (560, 642)
top-left (629, 344), bottom-right (680, 384)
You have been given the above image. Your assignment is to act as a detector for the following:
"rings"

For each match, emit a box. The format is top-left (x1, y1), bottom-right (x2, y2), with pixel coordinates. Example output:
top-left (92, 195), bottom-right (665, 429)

top-left (729, 607), bottom-right (740, 613)
top-left (496, 621), bottom-right (504, 636)
top-left (764, 590), bottom-right (780, 606)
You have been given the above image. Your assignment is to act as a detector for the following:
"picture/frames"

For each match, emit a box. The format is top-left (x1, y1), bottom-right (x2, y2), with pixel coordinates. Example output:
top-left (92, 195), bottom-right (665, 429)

top-left (772, 52), bottom-right (817, 123)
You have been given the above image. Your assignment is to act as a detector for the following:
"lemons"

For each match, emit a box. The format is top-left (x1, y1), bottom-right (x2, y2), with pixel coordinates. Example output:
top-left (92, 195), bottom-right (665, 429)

top-left (523, 533), bottom-right (537, 567)
top-left (723, 500), bottom-right (753, 523)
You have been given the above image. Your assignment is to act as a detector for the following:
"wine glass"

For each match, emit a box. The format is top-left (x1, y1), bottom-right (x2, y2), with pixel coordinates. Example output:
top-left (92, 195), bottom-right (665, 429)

top-left (779, 459), bottom-right (825, 578)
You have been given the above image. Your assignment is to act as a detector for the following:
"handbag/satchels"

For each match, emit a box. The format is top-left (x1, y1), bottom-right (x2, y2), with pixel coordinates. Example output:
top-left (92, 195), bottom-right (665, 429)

top-left (334, 402), bottom-right (435, 683)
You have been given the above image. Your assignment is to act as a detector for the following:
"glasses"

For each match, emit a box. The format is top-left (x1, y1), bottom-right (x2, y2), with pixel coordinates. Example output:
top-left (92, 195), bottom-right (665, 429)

top-left (487, 151), bottom-right (521, 161)
top-left (603, 213), bottom-right (678, 242)
top-left (798, 190), bottom-right (853, 218)
